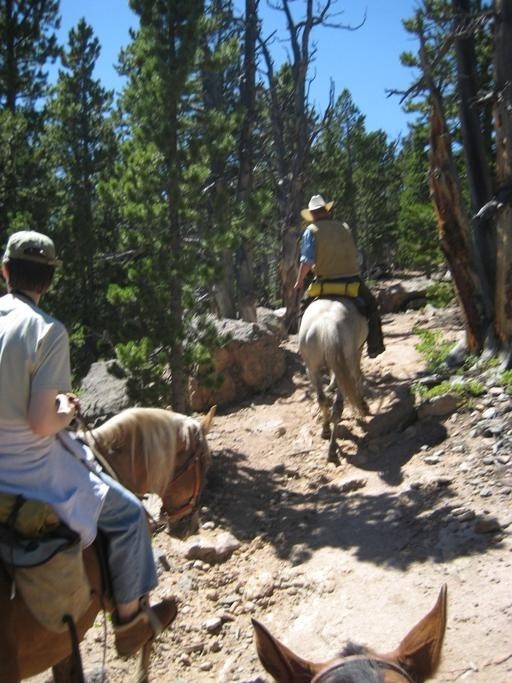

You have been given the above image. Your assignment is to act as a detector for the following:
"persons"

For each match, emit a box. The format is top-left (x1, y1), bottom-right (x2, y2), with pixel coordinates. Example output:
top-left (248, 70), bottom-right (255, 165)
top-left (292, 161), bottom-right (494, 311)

top-left (294, 194), bottom-right (386, 360)
top-left (1, 227), bottom-right (179, 659)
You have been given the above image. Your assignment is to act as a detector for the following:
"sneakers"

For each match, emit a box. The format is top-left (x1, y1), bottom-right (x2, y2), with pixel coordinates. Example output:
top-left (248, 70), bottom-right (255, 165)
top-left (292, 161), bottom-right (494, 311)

top-left (367, 344), bottom-right (385, 359)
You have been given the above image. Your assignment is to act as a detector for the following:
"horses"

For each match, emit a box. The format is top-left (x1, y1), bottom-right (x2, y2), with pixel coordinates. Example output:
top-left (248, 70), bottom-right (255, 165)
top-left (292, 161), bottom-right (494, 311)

top-left (296, 294), bottom-right (371, 465)
top-left (249, 584), bottom-right (450, 683)
top-left (0, 401), bottom-right (219, 683)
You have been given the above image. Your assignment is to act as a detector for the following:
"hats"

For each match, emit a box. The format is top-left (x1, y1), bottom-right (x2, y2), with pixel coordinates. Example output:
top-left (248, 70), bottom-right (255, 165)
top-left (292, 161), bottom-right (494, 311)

top-left (5, 230), bottom-right (58, 267)
top-left (301, 195), bottom-right (334, 221)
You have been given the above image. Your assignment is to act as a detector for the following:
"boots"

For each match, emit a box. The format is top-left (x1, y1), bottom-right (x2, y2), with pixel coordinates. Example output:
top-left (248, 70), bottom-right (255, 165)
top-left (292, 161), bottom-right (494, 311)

top-left (112, 595), bottom-right (178, 658)
